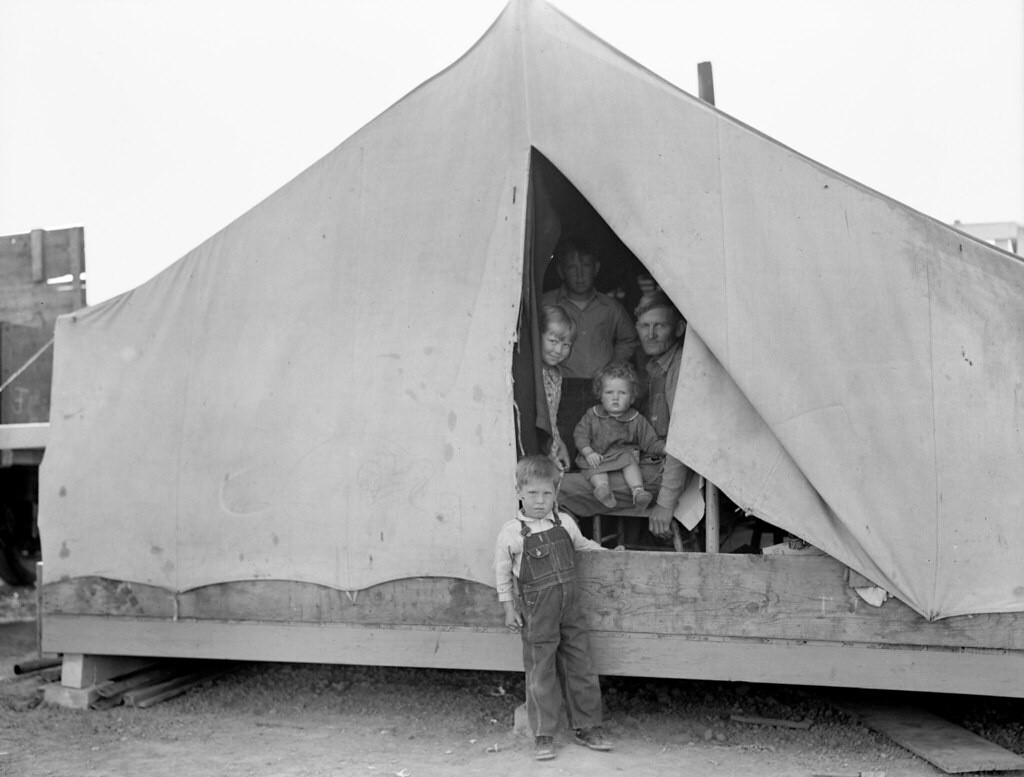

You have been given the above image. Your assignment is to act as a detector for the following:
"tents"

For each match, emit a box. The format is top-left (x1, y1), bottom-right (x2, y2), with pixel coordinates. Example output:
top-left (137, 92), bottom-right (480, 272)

top-left (35, 0), bottom-right (1024, 701)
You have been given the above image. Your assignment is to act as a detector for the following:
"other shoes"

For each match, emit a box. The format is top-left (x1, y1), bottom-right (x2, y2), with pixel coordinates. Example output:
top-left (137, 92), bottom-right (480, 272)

top-left (533, 736), bottom-right (556, 760)
top-left (634, 491), bottom-right (652, 516)
top-left (594, 483), bottom-right (616, 508)
top-left (573, 726), bottom-right (614, 749)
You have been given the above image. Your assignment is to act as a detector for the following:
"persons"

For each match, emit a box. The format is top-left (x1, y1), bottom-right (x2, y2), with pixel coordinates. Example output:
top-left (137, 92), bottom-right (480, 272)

top-left (495, 455), bottom-right (625, 760)
top-left (537, 238), bottom-right (696, 541)
